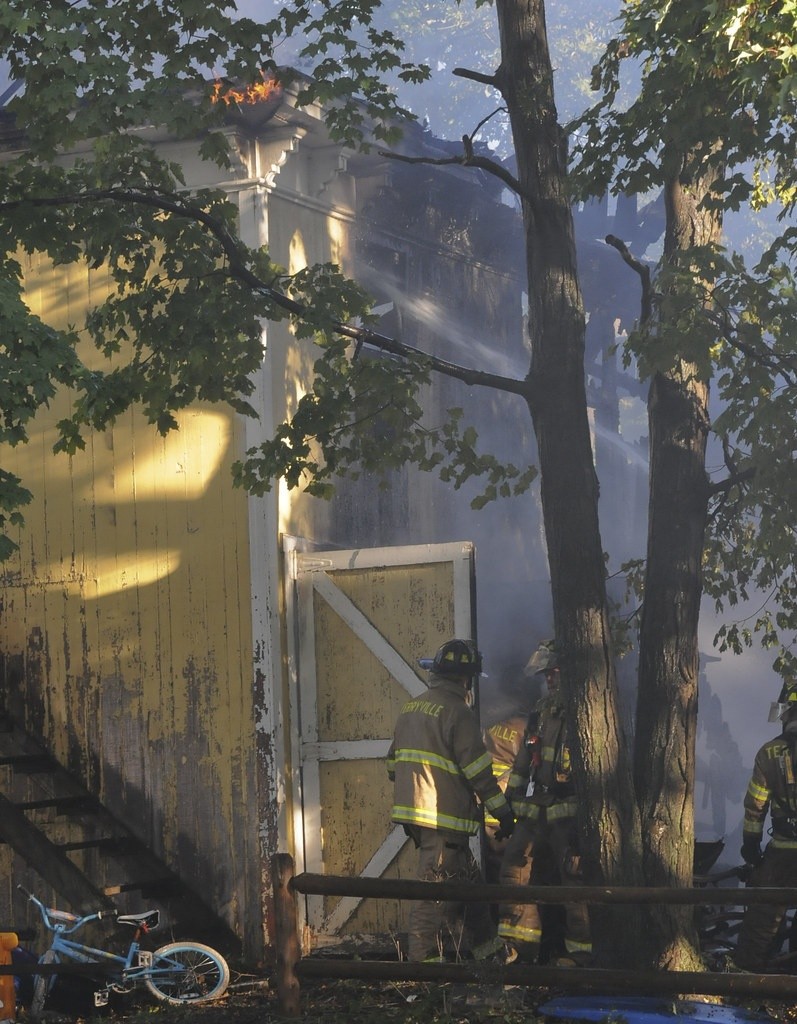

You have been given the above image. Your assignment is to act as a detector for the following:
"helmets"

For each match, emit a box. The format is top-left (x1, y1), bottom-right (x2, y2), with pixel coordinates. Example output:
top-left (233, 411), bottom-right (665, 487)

top-left (776, 680), bottom-right (797, 722)
top-left (418, 638), bottom-right (489, 680)
top-left (534, 640), bottom-right (559, 676)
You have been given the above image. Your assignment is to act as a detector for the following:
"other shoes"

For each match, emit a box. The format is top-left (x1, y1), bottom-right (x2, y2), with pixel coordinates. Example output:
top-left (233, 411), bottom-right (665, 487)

top-left (487, 945), bottom-right (519, 964)
top-left (714, 954), bottom-right (756, 974)
top-left (533, 953), bottom-right (579, 967)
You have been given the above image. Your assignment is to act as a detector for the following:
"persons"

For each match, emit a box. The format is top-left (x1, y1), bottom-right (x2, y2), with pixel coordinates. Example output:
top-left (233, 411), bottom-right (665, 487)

top-left (385, 639), bottom-right (588, 966)
top-left (733, 680), bottom-right (797, 975)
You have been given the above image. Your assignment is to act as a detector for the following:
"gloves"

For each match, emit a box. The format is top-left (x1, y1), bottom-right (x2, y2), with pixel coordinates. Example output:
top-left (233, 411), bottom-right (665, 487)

top-left (740, 840), bottom-right (762, 867)
top-left (494, 812), bottom-right (515, 842)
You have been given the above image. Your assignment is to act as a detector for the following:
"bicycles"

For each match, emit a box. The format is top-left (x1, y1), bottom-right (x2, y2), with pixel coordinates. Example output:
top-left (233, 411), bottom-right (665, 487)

top-left (16, 883), bottom-right (230, 1016)
